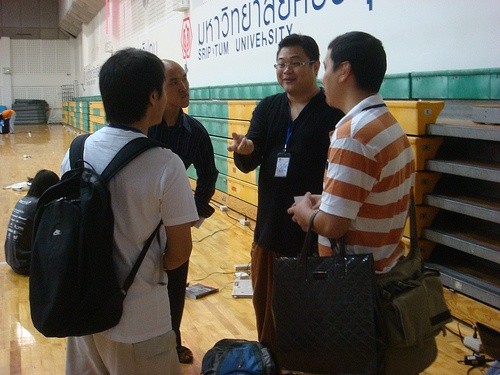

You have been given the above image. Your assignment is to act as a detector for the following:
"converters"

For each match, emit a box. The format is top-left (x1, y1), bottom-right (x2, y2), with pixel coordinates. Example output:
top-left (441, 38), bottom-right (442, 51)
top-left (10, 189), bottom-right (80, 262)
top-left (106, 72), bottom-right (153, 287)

top-left (463, 355), bottom-right (485, 366)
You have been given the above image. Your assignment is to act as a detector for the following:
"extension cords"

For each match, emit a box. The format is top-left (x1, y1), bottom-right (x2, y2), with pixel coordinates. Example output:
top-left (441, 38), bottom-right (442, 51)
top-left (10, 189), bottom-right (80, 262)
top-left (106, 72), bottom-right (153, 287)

top-left (463, 338), bottom-right (485, 353)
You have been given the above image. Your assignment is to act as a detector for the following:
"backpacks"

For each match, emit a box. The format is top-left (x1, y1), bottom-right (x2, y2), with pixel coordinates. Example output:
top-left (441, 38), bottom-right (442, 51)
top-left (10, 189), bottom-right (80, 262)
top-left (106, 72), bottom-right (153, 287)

top-left (200, 338), bottom-right (278, 375)
top-left (29, 132), bottom-right (163, 338)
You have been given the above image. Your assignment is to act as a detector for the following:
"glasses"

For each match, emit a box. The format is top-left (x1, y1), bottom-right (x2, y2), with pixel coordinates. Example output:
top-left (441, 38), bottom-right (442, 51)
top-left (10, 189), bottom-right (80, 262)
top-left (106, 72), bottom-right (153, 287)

top-left (274, 60), bottom-right (316, 71)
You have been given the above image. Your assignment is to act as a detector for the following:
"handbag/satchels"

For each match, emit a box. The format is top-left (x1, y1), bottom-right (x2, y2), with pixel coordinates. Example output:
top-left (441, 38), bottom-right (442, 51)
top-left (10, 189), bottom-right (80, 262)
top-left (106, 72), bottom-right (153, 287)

top-left (375, 248), bottom-right (454, 375)
top-left (271, 210), bottom-right (388, 375)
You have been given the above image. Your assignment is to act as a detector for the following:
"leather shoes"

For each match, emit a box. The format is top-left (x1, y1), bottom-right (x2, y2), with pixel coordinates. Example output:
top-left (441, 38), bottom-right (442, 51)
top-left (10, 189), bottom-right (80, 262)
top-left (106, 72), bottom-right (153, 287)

top-left (176, 345), bottom-right (194, 363)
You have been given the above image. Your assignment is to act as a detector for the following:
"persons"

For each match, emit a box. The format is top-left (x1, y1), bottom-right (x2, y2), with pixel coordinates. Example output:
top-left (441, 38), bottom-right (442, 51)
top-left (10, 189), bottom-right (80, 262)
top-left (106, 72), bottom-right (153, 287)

top-left (147, 58), bottom-right (218, 365)
top-left (229, 33), bottom-right (346, 374)
top-left (288, 31), bottom-right (417, 375)
top-left (59, 47), bottom-right (201, 375)
